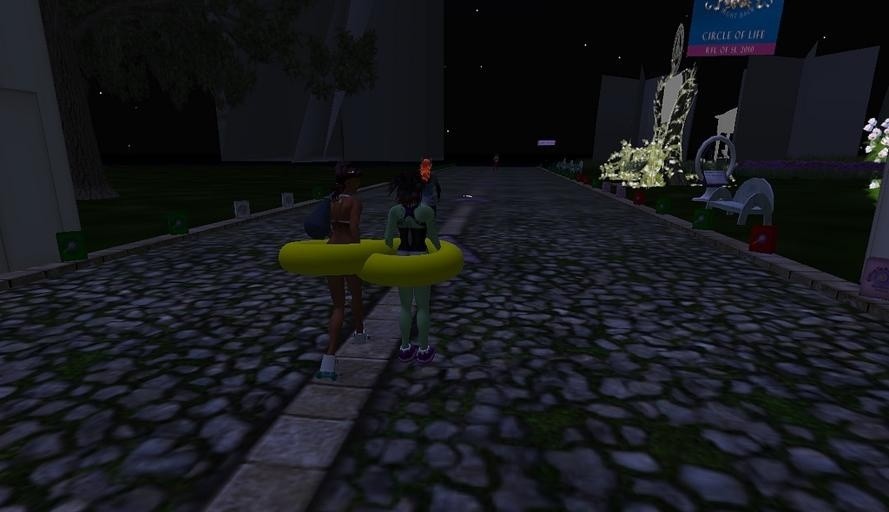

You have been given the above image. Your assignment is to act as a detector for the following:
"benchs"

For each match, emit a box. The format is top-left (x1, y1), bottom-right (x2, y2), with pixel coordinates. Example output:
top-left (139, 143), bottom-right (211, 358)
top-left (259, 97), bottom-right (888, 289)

top-left (705, 178), bottom-right (774, 226)
